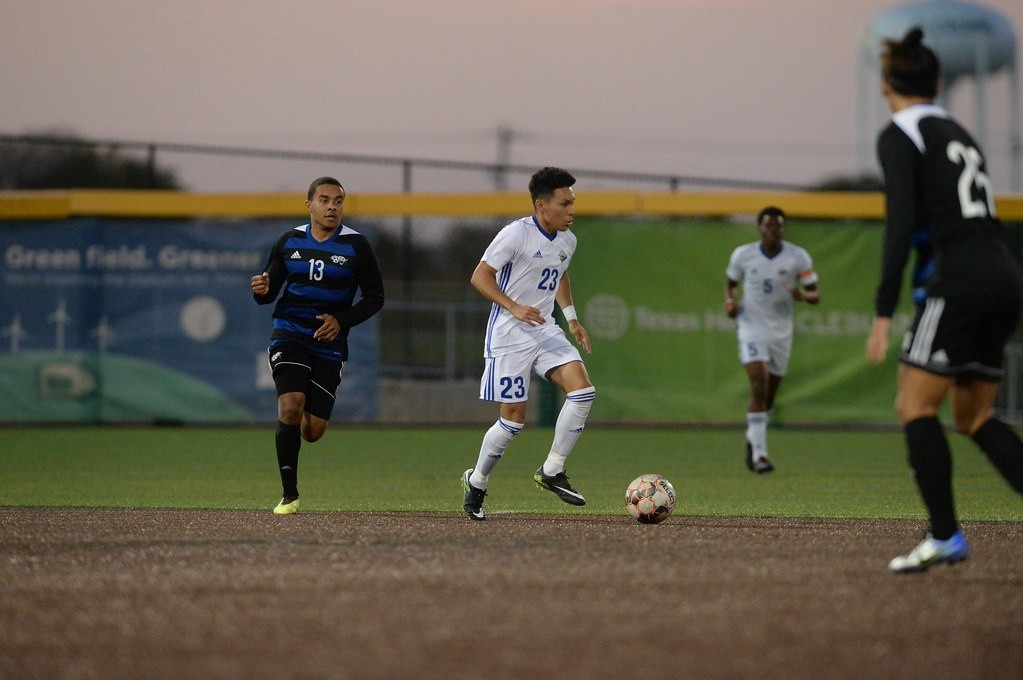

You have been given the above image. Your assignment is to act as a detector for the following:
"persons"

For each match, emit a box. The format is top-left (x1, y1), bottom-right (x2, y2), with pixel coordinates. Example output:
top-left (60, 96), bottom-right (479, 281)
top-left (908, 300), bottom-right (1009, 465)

top-left (865, 22), bottom-right (1023, 575)
top-left (724, 205), bottom-right (820, 474)
top-left (250, 176), bottom-right (387, 515)
top-left (459, 166), bottom-right (596, 521)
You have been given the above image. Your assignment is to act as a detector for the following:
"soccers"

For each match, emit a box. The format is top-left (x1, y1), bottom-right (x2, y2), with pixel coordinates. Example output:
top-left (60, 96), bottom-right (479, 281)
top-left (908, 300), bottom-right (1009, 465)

top-left (625, 473), bottom-right (677, 526)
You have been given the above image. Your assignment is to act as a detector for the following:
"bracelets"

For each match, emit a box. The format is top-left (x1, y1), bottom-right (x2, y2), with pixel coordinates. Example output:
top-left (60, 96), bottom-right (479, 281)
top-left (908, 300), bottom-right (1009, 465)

top-left (562, 304), bottom-right (578, 321)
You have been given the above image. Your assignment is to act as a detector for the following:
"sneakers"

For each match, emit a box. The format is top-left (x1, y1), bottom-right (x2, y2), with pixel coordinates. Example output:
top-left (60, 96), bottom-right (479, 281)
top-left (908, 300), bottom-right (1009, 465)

top-left (272, 496), bottom-right (300, 514)
top-left (745, 442), bottom-right (774, 475)
top-left (460, 469), bottom-right (488, 521)
top-left (533, 465), bottom-right (587, 506)
top-left (887, 530), bottom-right (971, 575)
